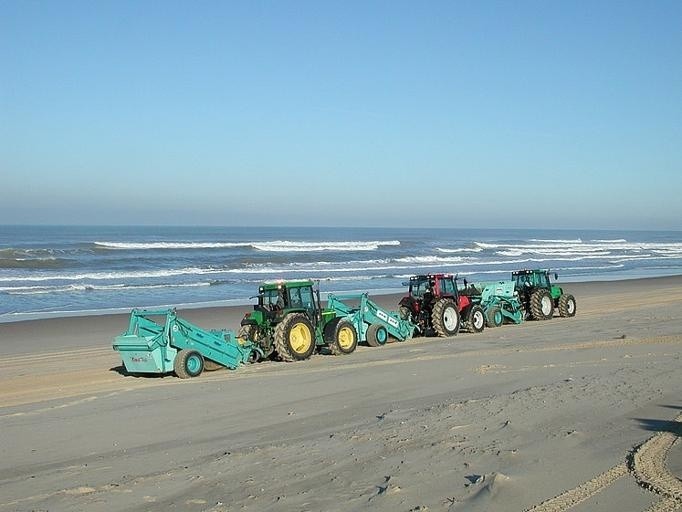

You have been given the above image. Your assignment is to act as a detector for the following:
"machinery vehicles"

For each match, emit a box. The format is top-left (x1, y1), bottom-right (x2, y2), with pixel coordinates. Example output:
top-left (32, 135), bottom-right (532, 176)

top-left (111, 278), bottom-right (357, 379)
top-left (473, 271), bottom-right (575, 326)
top-left (328, 273), bottom-right (485, 347)
